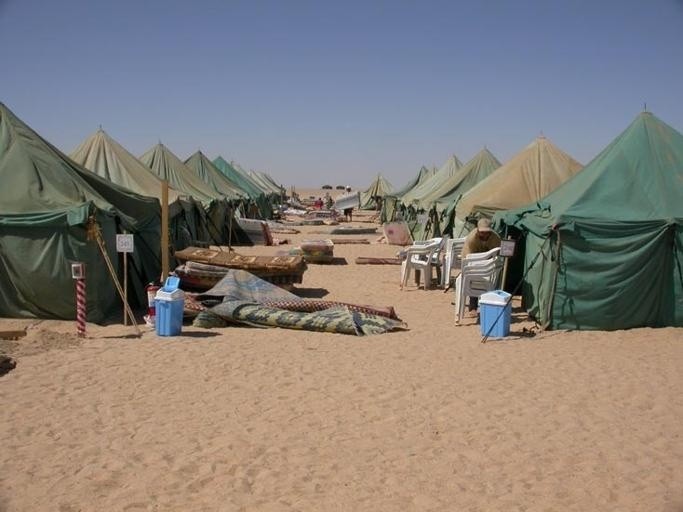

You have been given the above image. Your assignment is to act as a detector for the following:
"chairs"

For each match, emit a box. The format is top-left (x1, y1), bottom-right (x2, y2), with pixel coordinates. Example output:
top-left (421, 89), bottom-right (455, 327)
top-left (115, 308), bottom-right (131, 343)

top-left (400, 237), bottom-right (503, 326)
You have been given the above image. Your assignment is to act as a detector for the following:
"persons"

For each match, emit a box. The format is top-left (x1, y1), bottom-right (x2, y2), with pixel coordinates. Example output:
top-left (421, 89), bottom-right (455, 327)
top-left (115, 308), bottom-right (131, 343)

top-left (341, 185), bottom-right (353, 222)
top-left (323, 192), bottom-right (331, 201)
top-left (314, 197), bottom-right (324, 209)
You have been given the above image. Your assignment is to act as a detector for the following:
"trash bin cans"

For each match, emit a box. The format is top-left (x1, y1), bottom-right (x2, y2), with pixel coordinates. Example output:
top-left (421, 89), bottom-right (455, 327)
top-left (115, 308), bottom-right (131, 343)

top-left (479, 290), bottom-right (512, 337)
top-left (154, 276), bottom-right (185, 336)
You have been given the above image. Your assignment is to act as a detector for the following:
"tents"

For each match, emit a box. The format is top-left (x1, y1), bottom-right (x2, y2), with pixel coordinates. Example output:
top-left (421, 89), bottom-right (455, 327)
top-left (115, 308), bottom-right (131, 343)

top-left (0, 100), bottom-right (290, 327)
top-left (362, 96), bottom-right (682, 330)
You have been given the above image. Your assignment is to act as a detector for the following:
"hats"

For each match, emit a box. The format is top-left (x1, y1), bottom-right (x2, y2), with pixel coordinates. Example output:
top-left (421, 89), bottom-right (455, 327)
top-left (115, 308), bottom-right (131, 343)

top-left (477, 217), bottom-right (494, 234)
